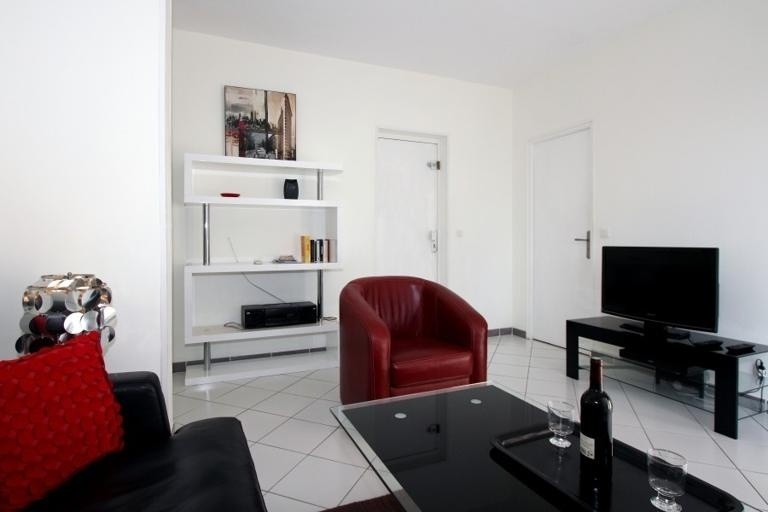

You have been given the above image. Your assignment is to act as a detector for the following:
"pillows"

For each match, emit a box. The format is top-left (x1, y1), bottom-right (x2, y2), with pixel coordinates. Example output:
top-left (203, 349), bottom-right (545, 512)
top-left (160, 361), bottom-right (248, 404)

top-left (0, 327), bottom-right (125, 512)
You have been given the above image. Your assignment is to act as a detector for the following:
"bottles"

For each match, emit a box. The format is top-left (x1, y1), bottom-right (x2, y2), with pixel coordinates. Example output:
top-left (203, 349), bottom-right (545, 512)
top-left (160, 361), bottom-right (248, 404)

top-left (577, 480), bottom-right (612, 510)
top-left (580, 358), bottom-right (614, 487)
top-left (283, 177), bottom-right (300, 199)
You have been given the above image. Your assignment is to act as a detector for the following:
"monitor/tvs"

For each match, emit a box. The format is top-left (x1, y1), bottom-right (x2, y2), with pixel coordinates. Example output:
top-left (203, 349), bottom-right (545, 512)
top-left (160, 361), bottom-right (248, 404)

top-left (602, 246), bottom-right (719, 340)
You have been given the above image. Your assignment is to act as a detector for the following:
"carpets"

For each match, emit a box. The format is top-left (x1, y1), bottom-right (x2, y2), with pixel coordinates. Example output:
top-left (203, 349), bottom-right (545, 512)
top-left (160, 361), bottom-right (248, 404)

top-left (318, 491), bottom-right (406, 512)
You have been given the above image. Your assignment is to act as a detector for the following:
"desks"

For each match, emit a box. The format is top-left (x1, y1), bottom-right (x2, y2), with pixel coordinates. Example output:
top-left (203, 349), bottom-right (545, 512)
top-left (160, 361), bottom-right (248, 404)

top-left (563, 315), bottom-right (767, 441)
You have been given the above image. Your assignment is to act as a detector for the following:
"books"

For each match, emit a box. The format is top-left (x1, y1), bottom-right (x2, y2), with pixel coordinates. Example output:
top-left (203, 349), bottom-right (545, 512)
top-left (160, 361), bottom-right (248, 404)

top-left (301, 235), bottom-right (329, 263)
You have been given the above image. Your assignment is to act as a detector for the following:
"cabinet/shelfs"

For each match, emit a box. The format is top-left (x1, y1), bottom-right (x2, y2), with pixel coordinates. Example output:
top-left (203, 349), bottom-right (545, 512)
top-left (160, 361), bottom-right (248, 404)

top-left (182, 152), bottom-right (346, 390)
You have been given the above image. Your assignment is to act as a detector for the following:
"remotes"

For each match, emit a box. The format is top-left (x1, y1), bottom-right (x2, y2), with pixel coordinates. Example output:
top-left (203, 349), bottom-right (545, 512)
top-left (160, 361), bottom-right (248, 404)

top-left (694, 339), bottom-right (724, 348)
top-left (725, 343), bottom-right (755, 352)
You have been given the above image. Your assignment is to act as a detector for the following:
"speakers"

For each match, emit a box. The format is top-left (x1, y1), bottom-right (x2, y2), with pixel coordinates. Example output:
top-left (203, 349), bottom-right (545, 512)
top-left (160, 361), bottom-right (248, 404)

top-left (283, 178), bottom-right (298, 199)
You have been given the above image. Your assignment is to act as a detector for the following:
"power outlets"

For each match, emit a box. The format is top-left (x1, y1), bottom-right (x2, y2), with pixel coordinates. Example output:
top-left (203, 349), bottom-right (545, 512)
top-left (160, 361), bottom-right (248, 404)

top-left (757, 363), bottom-right (767, 380)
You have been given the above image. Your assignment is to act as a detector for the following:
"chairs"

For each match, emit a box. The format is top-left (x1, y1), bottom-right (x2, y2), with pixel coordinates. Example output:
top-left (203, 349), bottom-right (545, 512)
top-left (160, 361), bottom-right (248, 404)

top-left (338, 274), bottom-right (489, 406)
top-left (6, 370), bottom-right (268, 512)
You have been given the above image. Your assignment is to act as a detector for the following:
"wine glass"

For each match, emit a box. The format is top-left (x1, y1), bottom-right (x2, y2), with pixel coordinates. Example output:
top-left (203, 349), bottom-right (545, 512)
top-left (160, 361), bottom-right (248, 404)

top-left (646, 447), bottom-right (689, 512)
top-left (546, 399), bottom-right (577, 449)
